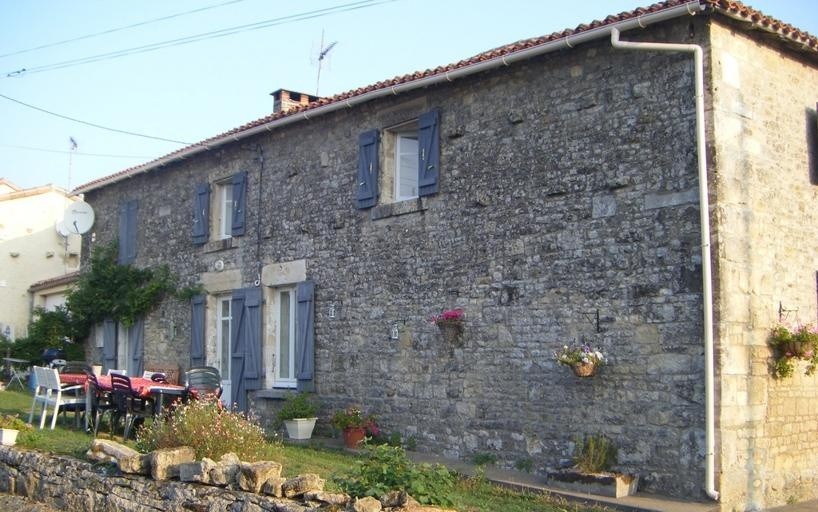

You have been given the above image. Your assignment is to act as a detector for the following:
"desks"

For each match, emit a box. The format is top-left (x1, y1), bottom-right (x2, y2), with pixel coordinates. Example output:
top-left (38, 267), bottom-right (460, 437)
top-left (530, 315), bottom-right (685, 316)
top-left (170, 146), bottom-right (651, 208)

top-left (2, 357), bottom-right (31, 391)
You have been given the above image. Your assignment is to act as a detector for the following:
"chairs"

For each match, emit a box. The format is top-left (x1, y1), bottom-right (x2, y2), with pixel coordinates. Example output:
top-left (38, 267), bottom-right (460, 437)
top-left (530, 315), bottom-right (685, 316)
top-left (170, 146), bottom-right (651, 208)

top-left (28, 361), bottom-right (227, 445)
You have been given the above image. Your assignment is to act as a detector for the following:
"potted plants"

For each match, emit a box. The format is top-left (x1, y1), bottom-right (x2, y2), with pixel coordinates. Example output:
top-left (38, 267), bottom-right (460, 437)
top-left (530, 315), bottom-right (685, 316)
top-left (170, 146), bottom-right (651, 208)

top-left (278, 390), bottom-right (325, 439)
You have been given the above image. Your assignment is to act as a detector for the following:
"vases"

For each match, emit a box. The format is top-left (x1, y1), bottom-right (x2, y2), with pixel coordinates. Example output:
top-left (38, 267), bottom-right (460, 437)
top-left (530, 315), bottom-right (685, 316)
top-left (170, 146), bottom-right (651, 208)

top-left (567, 360), bottom-right (597, 376)
top-left (786, 340), bottom-right (813, 359)
top-left (340, 426), bottom-right (367, 447)
top-left (436, 322), bottom-right (461, 342)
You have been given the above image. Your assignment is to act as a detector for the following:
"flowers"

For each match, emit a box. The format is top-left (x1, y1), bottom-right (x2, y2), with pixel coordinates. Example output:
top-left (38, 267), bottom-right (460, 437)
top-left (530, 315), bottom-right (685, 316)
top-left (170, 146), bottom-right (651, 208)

top-left (769, 304), bottom-right (818, 379)
top-left (332, 404), bottom-right (382, 437)
top-left (551, 335), bottom-right (608, 367)
top-left (432, 308), bottom-right (462, 326)
